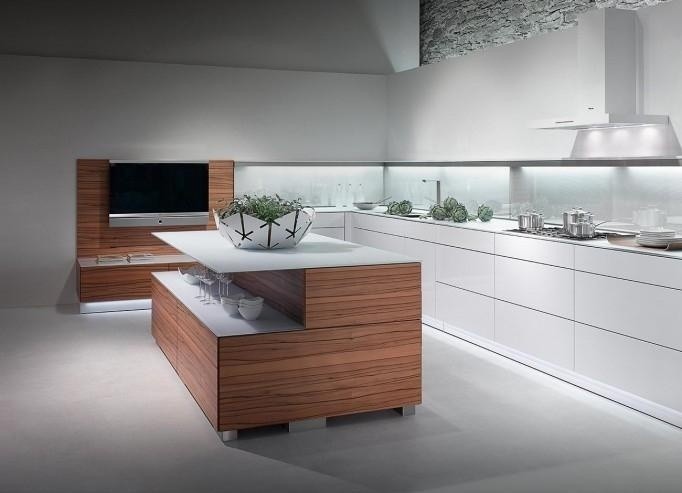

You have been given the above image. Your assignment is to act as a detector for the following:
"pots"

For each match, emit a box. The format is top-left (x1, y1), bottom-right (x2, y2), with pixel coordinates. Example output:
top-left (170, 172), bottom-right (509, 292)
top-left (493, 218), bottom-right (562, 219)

top-left (353, 195), bottom-right (392, 209)
top-left (517, 210), bottom-right (545, 231)
top-left (564, 207), bottom-right (594, 232)
top-left (567, 217), bottom-right (610, 240)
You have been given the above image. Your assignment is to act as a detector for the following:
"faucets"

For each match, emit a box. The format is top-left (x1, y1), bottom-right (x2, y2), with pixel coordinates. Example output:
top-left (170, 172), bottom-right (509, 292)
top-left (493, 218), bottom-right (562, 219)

top-left (422, 178), bottom-right (440, 206)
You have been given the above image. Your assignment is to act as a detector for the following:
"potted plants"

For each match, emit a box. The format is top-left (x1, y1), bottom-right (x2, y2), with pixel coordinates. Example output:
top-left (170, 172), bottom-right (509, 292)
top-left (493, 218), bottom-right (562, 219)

top-left (212, 194), bottom-right (315, 251)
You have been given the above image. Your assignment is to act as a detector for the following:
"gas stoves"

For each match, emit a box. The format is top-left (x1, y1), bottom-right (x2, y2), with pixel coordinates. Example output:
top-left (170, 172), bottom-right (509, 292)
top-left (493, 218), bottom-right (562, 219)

top-left (508, 227), bottom-right (617, 244)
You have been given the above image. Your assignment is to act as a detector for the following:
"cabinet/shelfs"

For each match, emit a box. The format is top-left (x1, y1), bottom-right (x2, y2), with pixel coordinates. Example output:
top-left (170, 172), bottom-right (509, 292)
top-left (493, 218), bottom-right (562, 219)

top-left (574, 246), bottom-right (681, 432)
top-left (309, 209), bottom-right (344, 239)
top-left (492, 232), bottom-right (573, 387)
top-left (76, 255), bottom-right (197, 314)
top-left (351, 215), bottom-right (492, 353)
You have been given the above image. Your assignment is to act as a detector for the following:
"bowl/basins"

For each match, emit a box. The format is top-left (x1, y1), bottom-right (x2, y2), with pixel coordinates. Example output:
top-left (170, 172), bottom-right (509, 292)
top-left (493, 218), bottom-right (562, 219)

top-left (178, 267), bottom-right (202, 286)
top-left (220, 293), bottom-right (265, 321)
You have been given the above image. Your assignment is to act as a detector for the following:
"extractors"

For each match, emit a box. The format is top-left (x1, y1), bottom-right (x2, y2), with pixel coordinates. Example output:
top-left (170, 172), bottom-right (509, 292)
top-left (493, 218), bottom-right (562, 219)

top-left (526, 8), bottom-right (671, 131)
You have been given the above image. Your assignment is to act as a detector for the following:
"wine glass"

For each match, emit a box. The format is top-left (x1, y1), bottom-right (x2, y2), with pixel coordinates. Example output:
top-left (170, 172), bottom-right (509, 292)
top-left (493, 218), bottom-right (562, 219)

top-left (188, 260), bottom-right (231, 306)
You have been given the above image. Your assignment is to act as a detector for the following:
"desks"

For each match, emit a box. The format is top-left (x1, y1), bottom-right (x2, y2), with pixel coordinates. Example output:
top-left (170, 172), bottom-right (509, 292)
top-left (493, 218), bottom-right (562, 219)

top-left (151, 227), bottom-right (422, 443)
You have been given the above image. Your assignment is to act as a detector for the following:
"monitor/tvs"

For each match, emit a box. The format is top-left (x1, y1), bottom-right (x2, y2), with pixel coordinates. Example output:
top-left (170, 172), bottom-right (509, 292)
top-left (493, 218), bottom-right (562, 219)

top-left (109, 159), bottom-right (208, 227)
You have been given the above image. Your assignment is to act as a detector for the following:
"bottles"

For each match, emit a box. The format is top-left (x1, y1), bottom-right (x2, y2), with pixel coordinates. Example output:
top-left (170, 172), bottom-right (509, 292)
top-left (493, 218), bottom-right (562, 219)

top-left (335, 182), bottom-right (364, 209)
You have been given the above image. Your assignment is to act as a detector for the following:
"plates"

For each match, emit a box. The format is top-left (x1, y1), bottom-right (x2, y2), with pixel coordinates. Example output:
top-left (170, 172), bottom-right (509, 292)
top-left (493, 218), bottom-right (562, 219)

top-left (636, 230), bottom-right (681, 249)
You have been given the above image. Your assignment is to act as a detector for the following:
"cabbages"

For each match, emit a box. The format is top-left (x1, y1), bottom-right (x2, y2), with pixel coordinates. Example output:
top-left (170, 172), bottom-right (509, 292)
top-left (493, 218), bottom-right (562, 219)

top-left (444, 196), bottom-right (457, 217)
top-left (478, 204), bottom-right (494, 222)
top-left (452, 205), bottom-right (468, 222)
top-left (431, 204), bottom-right (446, 221)
top-left (386, 200), bottom-right (413, 215)
